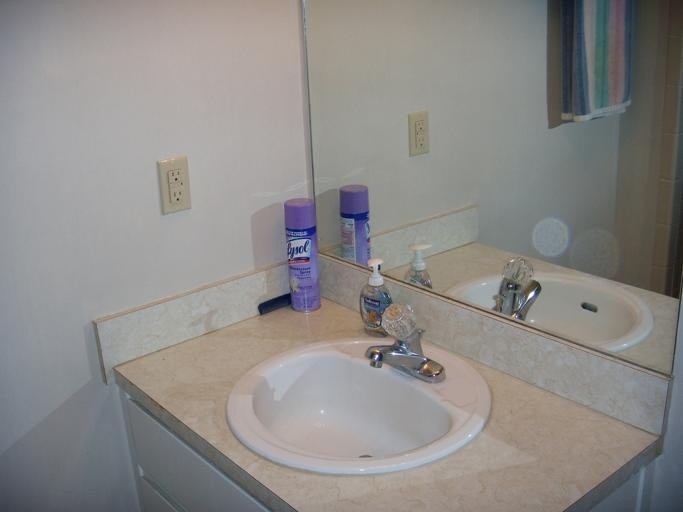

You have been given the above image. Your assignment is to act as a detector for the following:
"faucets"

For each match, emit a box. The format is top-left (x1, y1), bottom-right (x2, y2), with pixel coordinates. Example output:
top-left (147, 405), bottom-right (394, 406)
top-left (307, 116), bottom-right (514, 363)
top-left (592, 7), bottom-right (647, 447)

top-left (364, 303), bottom-right (446, 384)
top-left (491, 256), bottom-right (542, 323)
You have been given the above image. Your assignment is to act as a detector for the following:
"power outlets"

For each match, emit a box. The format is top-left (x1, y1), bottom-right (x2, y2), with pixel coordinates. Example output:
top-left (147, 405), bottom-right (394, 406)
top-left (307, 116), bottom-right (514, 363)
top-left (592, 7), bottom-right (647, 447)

top-left (407, 111), bottom-right (431, 157)
top-left (157, 157), bottom-right (192, 216)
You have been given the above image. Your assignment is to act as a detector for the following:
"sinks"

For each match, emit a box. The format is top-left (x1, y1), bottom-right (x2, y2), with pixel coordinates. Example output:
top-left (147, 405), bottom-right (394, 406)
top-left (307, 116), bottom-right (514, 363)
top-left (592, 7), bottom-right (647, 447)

top-left (442, 271), bottom-right (655, 354)
top-left (225, 338), bottom-right (493, 476)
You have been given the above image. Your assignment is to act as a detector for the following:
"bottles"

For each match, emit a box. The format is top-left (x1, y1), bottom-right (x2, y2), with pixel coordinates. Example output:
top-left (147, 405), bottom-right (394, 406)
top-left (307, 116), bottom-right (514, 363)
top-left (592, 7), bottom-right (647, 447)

top-left (283, 197), bottom-right (322, 312)
top-left (340, 184), bottom-right (370, 267)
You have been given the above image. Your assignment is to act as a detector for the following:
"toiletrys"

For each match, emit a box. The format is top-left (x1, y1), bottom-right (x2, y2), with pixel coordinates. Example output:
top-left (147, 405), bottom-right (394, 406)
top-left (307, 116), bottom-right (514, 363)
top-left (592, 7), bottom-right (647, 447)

top-left (358, 257), bottom-right (393, 334)
top-left (403, 243), bottom-right (435, 291)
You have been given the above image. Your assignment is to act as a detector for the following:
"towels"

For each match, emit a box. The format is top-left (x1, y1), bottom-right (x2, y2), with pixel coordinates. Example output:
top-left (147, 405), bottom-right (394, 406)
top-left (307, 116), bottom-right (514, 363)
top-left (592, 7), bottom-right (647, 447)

top-left (559, 2), bottom-right (636, 122)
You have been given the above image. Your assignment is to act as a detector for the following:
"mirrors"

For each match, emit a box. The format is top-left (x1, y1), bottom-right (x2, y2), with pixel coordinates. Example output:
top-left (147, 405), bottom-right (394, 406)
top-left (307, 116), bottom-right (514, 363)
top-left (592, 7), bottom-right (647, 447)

top-left (300, 3), bottom-right (681, 436)
